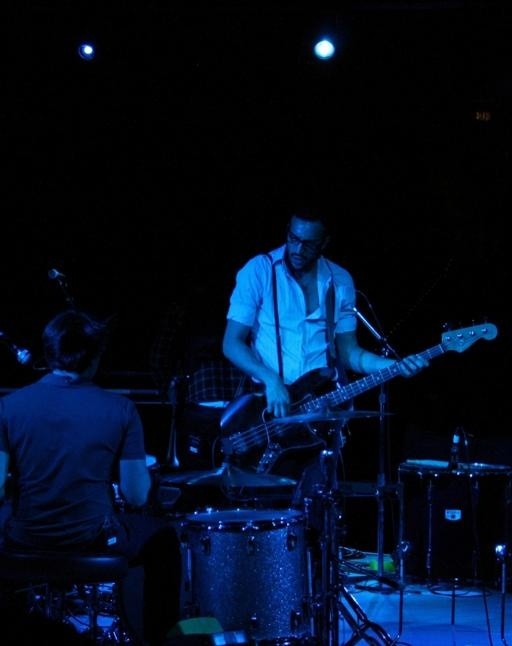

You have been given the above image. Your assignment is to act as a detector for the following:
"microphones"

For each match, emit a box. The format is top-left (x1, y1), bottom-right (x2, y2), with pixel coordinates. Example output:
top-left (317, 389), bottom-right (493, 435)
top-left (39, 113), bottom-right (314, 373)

top-left (351, 306), bottom-right (389, 345)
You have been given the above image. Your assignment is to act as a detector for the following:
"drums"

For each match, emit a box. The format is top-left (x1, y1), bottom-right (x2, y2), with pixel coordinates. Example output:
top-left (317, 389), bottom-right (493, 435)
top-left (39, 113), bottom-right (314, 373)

top-left (111, 453), bottom-right (166, 518)
top-left (187, 507), bottom-right (318, 646)
top-left (166, 504), bottom-right (218, 620)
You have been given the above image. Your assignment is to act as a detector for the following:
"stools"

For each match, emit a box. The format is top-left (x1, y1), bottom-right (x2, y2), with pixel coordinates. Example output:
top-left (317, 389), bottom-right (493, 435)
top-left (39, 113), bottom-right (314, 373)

top-left (10, 553), bottom-right (130, 646)
top-left (399, 466), bottom-right (512, 589)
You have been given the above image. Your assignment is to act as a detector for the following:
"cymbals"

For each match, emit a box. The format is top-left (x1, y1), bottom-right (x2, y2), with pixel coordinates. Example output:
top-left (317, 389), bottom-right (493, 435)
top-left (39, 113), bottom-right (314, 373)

top-left (164, 466), bottom-right (299, 486)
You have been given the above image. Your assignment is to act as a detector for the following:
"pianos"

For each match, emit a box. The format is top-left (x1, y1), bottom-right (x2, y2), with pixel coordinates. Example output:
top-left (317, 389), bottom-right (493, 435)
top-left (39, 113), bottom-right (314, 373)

top-left (0, 369), bottom-right (183, 406)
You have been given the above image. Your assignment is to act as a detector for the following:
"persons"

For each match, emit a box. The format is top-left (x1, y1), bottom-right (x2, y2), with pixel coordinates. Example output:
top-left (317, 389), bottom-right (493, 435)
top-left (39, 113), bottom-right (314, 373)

top-left (0, 310), bottom-right (160, 635)
top-left (222, 208), bottom-right (430, 419)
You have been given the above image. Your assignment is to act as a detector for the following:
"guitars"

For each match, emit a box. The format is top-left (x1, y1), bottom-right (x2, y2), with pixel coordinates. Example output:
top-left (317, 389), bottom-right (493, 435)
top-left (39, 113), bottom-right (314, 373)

top-left (218, 315), bottom-right (498, 474)
